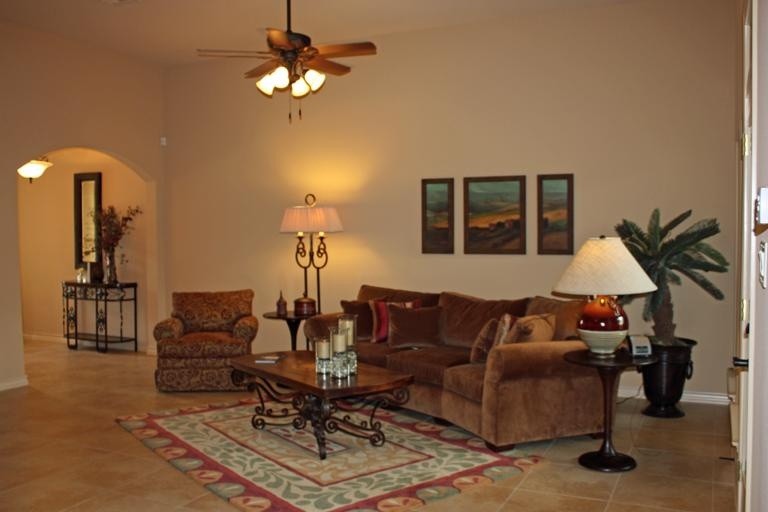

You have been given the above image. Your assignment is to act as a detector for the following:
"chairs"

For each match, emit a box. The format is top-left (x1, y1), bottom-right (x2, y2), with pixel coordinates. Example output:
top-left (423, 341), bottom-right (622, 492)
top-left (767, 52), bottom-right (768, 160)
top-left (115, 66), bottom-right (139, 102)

top-left (153, 288), bottom-right (259, 392)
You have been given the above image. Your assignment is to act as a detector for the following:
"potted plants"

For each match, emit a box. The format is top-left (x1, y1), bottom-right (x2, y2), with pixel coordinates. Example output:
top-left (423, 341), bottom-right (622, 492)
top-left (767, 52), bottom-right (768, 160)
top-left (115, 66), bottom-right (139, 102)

top-left (612, 207), bottom-right (730, 416)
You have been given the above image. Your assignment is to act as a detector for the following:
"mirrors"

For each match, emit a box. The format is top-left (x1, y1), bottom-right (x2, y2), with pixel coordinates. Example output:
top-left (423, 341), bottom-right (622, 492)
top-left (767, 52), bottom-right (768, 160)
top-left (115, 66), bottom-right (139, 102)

top-left (74, 172), bottom-right (101, 268)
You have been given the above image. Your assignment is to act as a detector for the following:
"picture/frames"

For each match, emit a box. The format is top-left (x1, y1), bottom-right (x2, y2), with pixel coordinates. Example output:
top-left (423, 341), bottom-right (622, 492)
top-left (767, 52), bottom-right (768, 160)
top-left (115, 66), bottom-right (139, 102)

top-left (462, 175), bottom-right (526, 254)
top-left (420, 177), bottom-right (455, 253)
top-left (536, 172), bottom-right (574, 255)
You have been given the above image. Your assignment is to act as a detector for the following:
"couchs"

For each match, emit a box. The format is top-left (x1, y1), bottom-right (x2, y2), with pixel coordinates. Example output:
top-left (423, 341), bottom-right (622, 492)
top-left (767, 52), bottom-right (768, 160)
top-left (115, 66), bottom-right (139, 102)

top-left (305, 284), bottom-right (633, 452)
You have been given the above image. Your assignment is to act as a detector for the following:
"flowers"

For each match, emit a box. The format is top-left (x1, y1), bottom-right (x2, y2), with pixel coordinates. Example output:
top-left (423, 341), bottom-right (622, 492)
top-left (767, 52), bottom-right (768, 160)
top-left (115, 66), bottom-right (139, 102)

top-left (80, 204), bottom-right (142, 262)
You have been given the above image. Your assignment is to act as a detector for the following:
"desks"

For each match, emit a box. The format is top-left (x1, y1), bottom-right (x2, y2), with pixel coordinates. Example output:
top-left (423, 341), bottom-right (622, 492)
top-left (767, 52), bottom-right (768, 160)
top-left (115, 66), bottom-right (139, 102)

top-left (60, 279), bottom-right (138, 353)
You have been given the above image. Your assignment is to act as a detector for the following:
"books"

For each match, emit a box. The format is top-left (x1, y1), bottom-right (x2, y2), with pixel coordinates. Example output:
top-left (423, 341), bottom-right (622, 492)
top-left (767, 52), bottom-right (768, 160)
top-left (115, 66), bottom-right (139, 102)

top-left (254, 355), bottom-right (282, 364)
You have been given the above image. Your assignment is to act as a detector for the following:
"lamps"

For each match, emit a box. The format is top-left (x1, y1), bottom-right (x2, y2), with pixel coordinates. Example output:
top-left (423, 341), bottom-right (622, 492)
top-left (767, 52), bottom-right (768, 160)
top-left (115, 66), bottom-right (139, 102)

top-left (278, 192), bottom-right (343, 350)
top-left (256, 50), bottom-right (326, 122)
top-left (17, 155), bottom-right (53, 183)
top-left (550, 235), bottom-right (658, 356)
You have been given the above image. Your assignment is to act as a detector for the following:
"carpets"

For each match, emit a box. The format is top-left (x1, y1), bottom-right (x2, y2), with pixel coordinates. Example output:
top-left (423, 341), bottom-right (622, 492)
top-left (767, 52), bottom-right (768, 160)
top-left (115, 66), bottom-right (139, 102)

top-left (114, 396), bottom-right (543, 512)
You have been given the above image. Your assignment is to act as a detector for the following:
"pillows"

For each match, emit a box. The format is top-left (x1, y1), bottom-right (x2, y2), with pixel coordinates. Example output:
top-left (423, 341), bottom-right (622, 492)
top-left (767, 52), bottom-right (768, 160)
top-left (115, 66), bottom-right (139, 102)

top-left (469, 312), bottom-right (556, 361)
top-left (341, 298), bottom-right (443, 347)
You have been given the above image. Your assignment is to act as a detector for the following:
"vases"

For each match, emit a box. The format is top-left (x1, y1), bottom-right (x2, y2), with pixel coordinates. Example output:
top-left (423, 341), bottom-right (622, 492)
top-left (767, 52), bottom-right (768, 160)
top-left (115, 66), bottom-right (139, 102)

top-left (106, 255), bottom-right (117, 286)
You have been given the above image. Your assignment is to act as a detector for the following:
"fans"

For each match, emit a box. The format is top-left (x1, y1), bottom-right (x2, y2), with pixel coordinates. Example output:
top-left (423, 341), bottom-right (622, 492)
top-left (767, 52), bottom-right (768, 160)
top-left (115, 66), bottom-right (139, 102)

top-left (195, 1), bottom-right (378, 78)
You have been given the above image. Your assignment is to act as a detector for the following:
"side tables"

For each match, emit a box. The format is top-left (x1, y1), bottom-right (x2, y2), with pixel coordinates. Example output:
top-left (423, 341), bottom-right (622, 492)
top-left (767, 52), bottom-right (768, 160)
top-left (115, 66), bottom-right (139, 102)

top-left (563, 349), bottom-right (658, 473)
top-left (262, 309), bottom-right (319, 351)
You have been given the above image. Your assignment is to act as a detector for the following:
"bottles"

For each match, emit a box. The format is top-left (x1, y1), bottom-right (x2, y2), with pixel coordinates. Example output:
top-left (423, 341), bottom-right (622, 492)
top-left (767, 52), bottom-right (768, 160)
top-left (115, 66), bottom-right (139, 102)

top-left (77, 272), bottom-right (86, 284)
